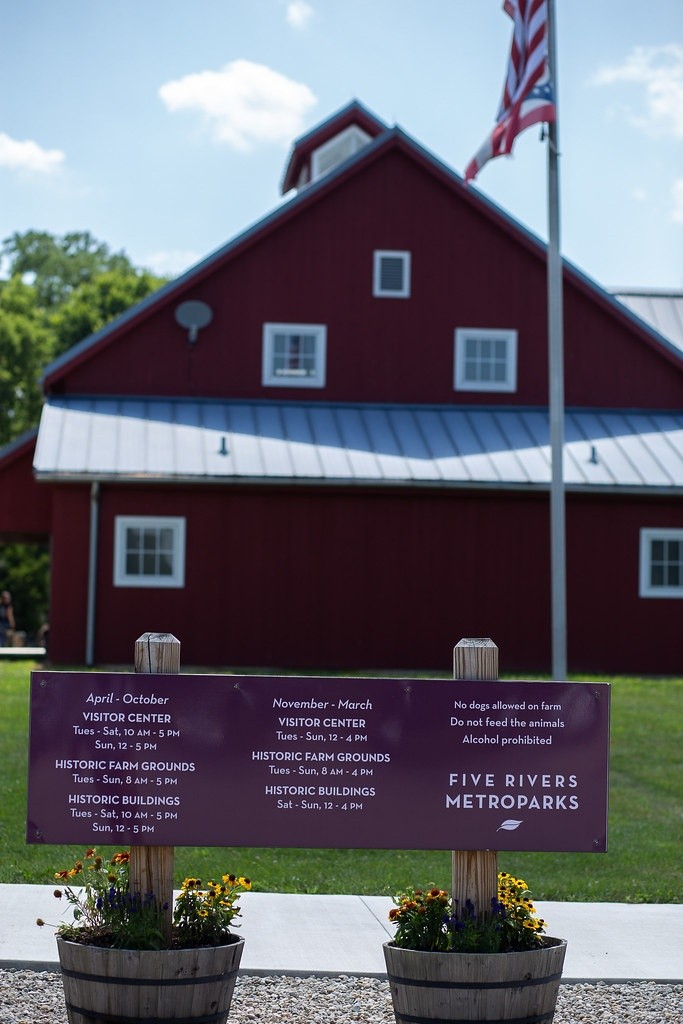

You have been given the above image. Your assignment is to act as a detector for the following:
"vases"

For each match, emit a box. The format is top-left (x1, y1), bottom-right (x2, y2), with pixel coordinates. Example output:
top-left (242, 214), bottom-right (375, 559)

top-left (382, 935), bottom-right (567, 1024)
top-left (56, 927), bottom-right (246, 1024)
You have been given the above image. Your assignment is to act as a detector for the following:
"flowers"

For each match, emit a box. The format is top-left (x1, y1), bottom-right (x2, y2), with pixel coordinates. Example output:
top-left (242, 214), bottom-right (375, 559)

top-left (388, 872), bottom-right (547, 953)
top-left (37, 848), bottom-right (251, 950)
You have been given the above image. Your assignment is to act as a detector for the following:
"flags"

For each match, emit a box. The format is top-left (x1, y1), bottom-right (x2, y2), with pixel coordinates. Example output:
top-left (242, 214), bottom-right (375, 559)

top-left (460, 0), bottom-right (556, 186)
